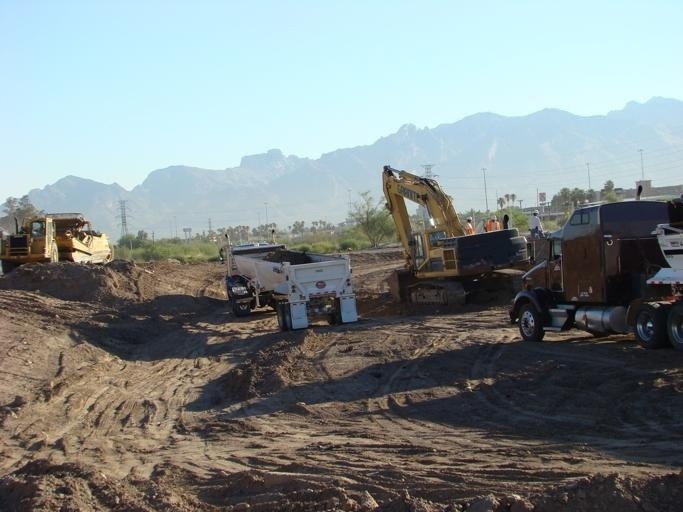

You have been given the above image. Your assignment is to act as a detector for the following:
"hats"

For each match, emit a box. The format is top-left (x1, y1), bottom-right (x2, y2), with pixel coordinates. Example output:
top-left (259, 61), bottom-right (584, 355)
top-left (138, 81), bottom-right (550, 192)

top-left (490, 215), bottom-right (498, 221)
top-left (467, 216), bottom-right (472, 221)
top-left (532, 209), bottom-right (539, 214)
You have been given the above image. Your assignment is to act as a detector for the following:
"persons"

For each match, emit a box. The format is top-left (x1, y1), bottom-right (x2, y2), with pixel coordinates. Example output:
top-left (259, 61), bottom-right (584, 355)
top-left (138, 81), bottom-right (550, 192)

top-left (464, 217), bottom-right (474, 235)
top-left (219, 248), bottom-right (225, 264)
top-left (486, 215), bottom-right (500, 232)
top-left (528, 210), bottom-right (546, 240)
top-left (475, 219), bottom-right (486, 233)
top-left (500, 214), bottom-right (512, 229)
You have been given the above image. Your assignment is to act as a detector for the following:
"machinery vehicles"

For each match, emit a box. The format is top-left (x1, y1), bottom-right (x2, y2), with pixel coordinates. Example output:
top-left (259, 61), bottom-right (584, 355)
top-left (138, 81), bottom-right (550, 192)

top-left (373, 163), bottom-right (534, 308)
top-left (0, 209), bottom-right (114, 277)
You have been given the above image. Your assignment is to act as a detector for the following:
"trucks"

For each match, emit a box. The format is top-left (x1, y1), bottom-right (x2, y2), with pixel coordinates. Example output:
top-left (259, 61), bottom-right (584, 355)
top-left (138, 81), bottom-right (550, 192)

top-left (505, 184), bottom-right (681, 352)
top-left (216, 228), bottom-right (359, 334)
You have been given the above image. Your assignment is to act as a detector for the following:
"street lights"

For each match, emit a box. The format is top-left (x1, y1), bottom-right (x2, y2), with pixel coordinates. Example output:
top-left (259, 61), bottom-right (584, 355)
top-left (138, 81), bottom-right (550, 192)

top-left (586, 162), bottom-right (590, 188)
top-left (636, 148), bottom-right (644, 180)
top-left (346, 188), bottom-right (352, 210)
top-left (263, 201), bottom-right (268, 224)
top-left (480, 166), bottom-right (489, 212)
